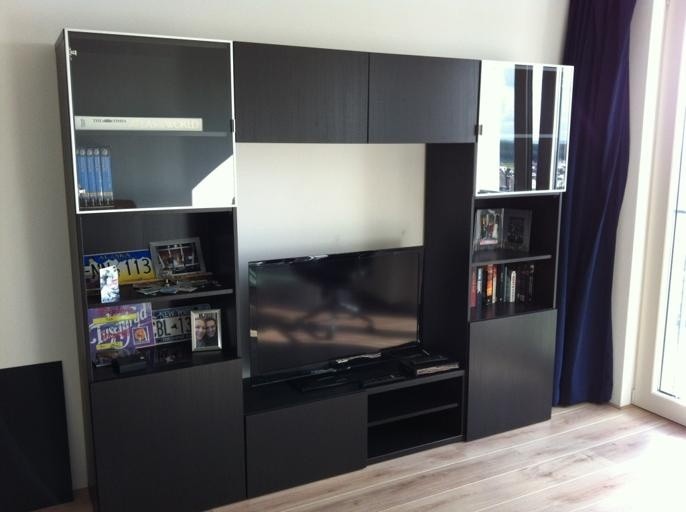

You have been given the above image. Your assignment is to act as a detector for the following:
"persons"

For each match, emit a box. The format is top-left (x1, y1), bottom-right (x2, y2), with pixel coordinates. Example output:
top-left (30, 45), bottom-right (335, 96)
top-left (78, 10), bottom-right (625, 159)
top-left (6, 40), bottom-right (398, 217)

top-left (482, 220), bottom-right (499, 242)
top-left (101, 272), bottom-right (119, 304)
top-left (195, 316), bottom-right (218, 347)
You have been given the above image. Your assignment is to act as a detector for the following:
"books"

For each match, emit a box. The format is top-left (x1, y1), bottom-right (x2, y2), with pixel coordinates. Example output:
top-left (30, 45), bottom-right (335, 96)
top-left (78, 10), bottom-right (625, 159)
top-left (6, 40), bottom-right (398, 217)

top-left (75, 145), bottom-right (117, 210)
top-left (470, 260), bottom-right (536, 308)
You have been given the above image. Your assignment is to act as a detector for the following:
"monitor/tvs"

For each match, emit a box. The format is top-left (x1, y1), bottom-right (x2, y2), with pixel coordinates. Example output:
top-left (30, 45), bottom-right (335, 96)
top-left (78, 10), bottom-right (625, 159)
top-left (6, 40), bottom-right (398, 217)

top-left (248, 246), bottom-right (424, 393)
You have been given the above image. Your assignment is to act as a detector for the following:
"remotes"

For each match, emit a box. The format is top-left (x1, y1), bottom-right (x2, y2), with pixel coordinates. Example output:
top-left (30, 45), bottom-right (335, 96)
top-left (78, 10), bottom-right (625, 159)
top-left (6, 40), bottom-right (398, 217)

top-left (359, 373), bottom-right (396, 389)
top-left (411, 354), bottom-right (448, 366)
